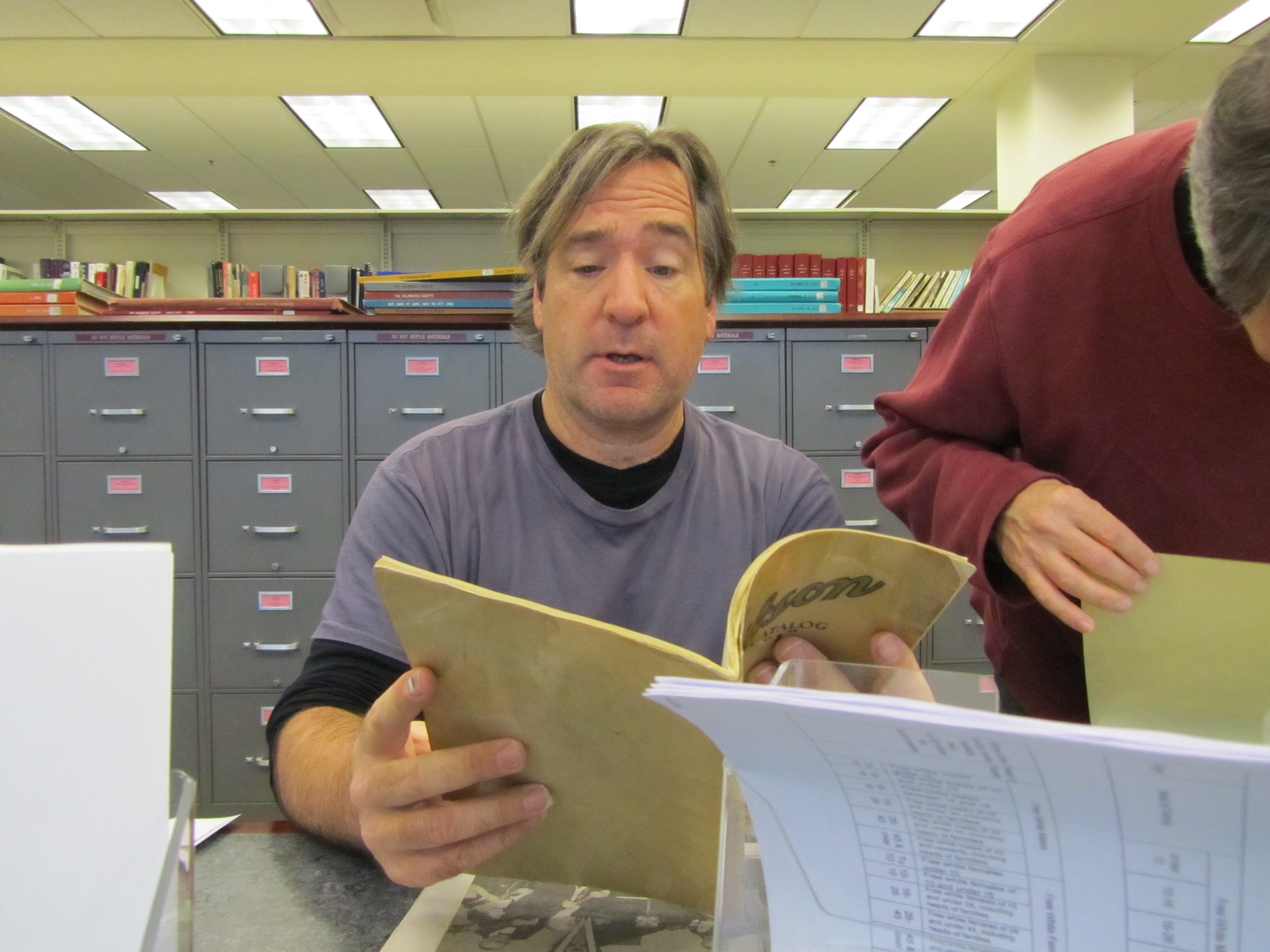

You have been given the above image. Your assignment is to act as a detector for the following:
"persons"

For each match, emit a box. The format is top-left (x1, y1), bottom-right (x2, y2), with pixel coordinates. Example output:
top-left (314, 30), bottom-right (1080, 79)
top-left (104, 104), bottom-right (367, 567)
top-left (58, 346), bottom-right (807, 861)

top-left (461, 874), bottom-right (714, 950)
top-left (864, 41), bottom-right (1270, 721)
top-left (264, 124), bottom-right (939, 891)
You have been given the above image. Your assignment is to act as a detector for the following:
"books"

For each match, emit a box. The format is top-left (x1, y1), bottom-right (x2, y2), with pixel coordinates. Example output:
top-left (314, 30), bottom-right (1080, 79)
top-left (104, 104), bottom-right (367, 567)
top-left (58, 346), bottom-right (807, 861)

top-left (369, 530), bottom-right (1270, 952)
top-left (717, 254), bottom-right (972, 314)
top-left (0, 259), bottom-right (537, 316)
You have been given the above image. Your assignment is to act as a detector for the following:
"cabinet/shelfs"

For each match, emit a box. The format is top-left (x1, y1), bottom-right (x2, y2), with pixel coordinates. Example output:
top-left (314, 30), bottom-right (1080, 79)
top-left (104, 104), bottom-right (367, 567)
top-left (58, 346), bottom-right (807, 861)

top-left (0, 331), bottom-right (1001, 822)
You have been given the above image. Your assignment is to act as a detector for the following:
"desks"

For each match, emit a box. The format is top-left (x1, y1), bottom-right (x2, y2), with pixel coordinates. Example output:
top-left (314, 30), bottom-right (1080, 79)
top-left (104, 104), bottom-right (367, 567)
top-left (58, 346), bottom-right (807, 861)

top-left (194, 821), bottom-right (423, 952)
